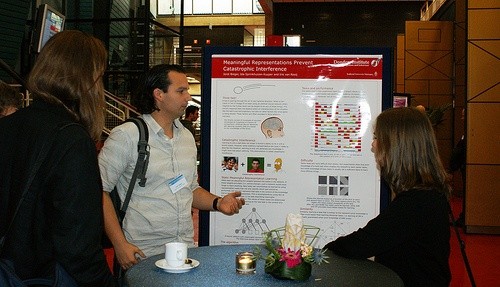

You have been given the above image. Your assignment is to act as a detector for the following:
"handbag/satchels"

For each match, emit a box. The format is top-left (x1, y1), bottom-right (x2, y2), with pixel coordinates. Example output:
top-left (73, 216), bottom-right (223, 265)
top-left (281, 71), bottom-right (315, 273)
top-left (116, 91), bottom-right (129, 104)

top-left (100, 223), bottom-right (118, 278)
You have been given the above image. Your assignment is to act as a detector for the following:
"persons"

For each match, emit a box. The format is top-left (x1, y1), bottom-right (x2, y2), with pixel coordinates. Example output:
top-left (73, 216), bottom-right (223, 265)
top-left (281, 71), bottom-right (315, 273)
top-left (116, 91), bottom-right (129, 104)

top-left (324, 107), bottom-right (453, 287)
top-left (97, 64), bottom-right (244, 287)
top-left (223, 157), bottom-right (238, 171)
top-left (0, 30), bottom-right (119, 287)
top-left (0, 80), bottom-right (22, 119)
top-left (262, 117), bottom-right (285, 139)
top-left (248, 158), bottom-right (263, 172)
top-left (180, 105), bottom-right (199, 137)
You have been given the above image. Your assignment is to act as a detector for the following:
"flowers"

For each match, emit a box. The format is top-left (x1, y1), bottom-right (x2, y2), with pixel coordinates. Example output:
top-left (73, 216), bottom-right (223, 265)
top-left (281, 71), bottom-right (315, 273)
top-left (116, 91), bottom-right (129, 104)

top-left (253, 226), bottom-right (332, 268)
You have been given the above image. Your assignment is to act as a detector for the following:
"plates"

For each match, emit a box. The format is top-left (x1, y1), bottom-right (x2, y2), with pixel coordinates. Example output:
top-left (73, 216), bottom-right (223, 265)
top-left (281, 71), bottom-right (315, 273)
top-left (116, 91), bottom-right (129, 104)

top-left (154, 258), bottom-right (200, 273)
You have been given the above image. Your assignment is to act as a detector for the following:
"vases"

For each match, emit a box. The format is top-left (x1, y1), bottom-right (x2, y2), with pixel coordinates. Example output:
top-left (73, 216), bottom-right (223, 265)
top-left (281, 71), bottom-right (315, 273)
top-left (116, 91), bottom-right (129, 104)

top-left (266, 253), bottom-right (312, 280)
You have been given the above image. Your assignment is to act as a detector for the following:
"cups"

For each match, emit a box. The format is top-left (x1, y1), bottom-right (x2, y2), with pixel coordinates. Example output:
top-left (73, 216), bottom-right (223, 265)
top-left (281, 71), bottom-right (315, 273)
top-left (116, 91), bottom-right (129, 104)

top-left (236, 251), bottom-right (256, 274)
top-left (165, 242), bottom-right (187, 268)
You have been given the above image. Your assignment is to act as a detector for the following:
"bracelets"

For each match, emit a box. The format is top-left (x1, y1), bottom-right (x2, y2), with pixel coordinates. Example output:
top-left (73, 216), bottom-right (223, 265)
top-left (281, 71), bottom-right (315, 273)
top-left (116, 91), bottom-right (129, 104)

top-left (213, 197), bottom-right (222, 211)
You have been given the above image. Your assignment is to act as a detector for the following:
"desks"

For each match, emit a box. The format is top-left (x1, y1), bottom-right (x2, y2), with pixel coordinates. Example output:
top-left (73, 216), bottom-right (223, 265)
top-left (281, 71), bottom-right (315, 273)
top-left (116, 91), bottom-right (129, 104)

top-left (126, 244), bottom-right (404, 287)
